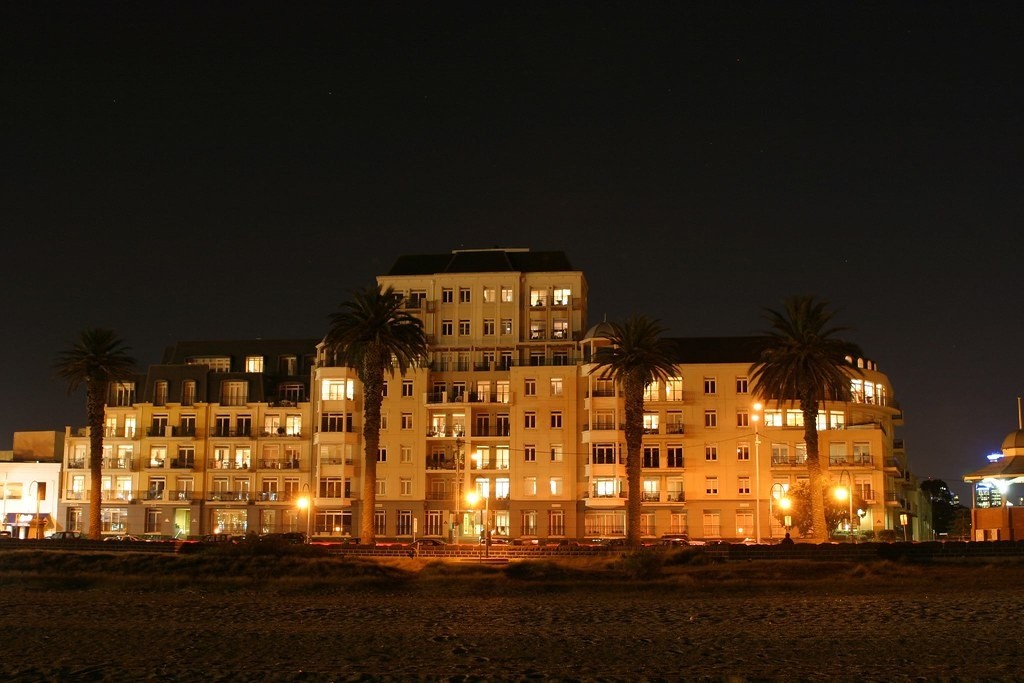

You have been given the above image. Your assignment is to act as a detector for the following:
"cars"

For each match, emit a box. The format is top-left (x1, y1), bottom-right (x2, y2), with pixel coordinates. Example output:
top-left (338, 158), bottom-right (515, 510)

top-left (598, 531), bottom-right (767, 547)
top-left (48, 528), bottom-right (310, 547)
top-left (407, 538), bottom-right (447, 547)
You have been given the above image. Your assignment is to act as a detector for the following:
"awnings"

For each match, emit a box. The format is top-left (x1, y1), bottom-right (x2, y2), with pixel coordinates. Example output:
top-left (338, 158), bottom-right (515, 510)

top-left (2, 513), bottom-right (51, 527)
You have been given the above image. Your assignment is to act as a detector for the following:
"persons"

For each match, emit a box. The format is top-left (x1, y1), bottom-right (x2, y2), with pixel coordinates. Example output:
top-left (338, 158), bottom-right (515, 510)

top-left (782, 533), bottom-right (794, 544)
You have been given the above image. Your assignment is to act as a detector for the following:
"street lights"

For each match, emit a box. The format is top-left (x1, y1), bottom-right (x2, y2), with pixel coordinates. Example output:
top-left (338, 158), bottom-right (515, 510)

top-left (297, 483), bottom-right (311, 545)
top-left (467, 474), bottom-right (491, 558)
top-left (25, 481), bottom-right (41, 539)
top-left (770, 482), bottom-right (788, 545)
top-left (835, 469), bottom-right (854, 543)
